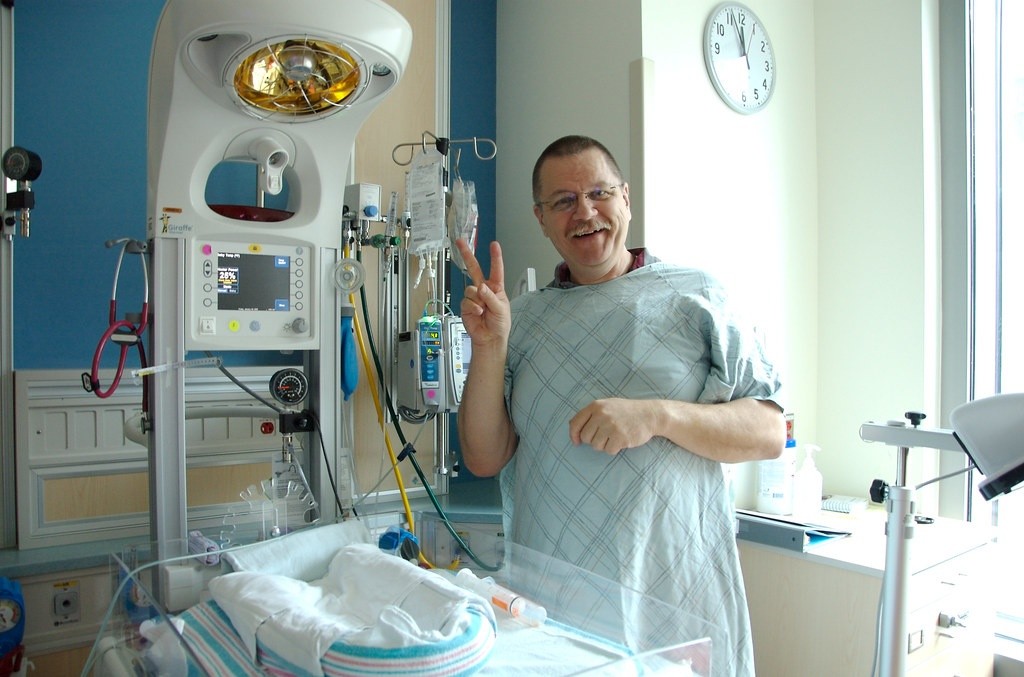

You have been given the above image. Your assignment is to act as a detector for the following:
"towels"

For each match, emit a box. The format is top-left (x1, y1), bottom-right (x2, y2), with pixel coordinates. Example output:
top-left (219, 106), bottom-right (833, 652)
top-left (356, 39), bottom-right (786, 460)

top-left (208, 541), bottom-right (497, 677)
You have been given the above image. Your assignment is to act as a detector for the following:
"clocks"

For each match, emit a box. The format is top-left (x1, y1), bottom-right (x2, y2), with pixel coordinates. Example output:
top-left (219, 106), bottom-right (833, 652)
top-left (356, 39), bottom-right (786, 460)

top-left (703, 1), bottom-right (777, 116)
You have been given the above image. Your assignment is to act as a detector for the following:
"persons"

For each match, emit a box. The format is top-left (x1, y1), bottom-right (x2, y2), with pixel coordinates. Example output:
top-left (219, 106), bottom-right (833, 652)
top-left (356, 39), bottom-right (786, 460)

top-left (456, 135), bottom-right (788, 677)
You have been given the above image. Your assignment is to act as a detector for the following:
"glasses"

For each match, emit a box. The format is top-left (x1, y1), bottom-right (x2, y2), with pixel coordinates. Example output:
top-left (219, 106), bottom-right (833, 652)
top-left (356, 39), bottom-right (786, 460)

top-left (537, 183), bottom-right (624, 210)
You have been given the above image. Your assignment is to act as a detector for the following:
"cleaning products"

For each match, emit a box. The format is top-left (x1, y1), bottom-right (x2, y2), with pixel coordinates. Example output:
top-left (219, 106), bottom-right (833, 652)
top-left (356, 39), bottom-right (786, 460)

top-left (794, 443), bottom-right (823, 519)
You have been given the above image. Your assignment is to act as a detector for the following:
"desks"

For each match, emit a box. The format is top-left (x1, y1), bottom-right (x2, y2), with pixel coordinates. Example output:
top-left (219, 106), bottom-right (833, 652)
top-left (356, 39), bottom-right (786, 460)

top-left (736, 497), bottom-right (1001, 677)
